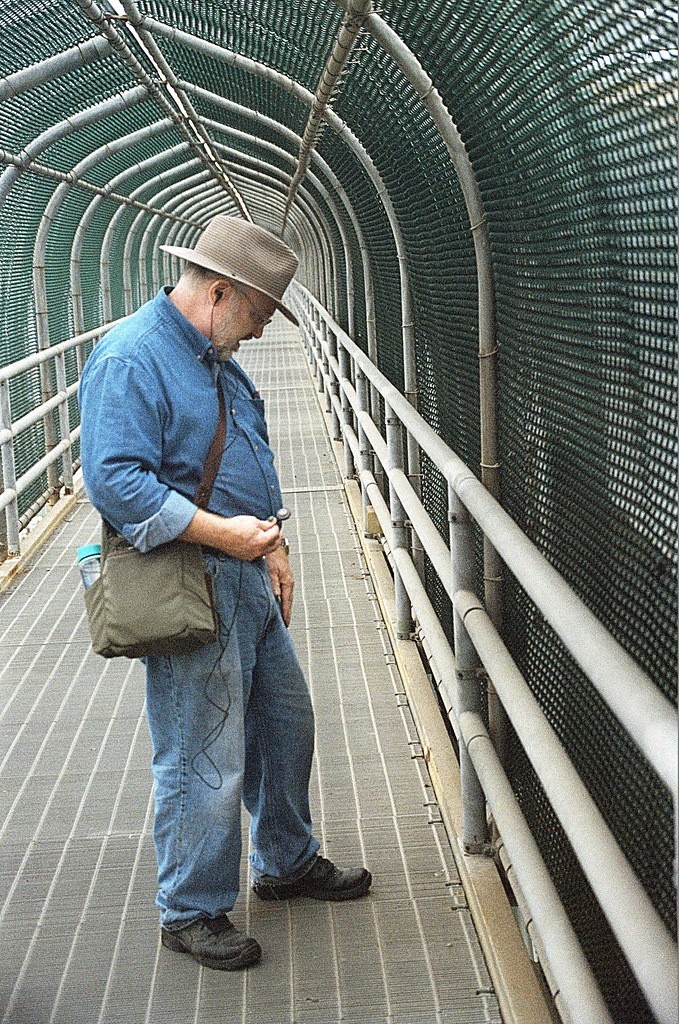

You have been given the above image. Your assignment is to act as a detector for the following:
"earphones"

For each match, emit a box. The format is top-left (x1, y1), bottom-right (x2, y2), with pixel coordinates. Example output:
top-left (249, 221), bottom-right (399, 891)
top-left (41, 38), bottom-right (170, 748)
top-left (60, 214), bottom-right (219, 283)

top-left (213, 290), bottom-right (223, 305)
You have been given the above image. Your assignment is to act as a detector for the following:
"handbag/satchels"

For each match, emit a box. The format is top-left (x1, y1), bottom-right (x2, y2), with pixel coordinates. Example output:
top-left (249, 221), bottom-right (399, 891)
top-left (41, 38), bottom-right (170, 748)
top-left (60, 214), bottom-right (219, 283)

top-left (82, 531), bottom-right (219, 662)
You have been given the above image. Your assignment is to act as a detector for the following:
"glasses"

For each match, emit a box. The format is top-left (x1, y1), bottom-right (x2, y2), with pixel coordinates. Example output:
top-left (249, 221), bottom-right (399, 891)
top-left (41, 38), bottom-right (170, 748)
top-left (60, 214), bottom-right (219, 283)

top-left (232, 283), bottom-right (273, 332)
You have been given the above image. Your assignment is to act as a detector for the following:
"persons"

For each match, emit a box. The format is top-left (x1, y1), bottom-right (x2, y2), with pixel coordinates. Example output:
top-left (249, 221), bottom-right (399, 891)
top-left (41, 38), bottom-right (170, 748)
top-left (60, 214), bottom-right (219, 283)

top-left (78, 215), bottom-right (372, 970)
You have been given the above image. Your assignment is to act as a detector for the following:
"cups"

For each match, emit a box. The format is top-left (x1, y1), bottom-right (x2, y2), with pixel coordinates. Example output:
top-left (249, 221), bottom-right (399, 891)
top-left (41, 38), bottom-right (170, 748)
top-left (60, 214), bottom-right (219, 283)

top-left (77, 545), bottom-right (102, 592)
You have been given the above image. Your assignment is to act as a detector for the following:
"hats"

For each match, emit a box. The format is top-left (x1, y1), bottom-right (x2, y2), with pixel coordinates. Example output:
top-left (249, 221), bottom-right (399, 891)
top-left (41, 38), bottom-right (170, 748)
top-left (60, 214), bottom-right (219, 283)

top-left (159, 214), bottom-right (304, 329)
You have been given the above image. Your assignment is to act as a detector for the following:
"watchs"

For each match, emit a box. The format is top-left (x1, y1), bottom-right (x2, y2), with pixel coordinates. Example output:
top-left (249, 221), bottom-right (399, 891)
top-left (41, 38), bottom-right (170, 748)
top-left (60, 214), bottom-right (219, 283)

top-left (281, 537), bottom-right (290, 555)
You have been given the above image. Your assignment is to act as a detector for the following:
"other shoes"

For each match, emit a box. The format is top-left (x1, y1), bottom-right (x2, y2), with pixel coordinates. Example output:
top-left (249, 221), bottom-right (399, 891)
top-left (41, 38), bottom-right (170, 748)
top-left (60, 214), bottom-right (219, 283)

top-left (250, 856), bottom-right (373, 903)
top-left (161, 912), bottom-right (262, 972)
